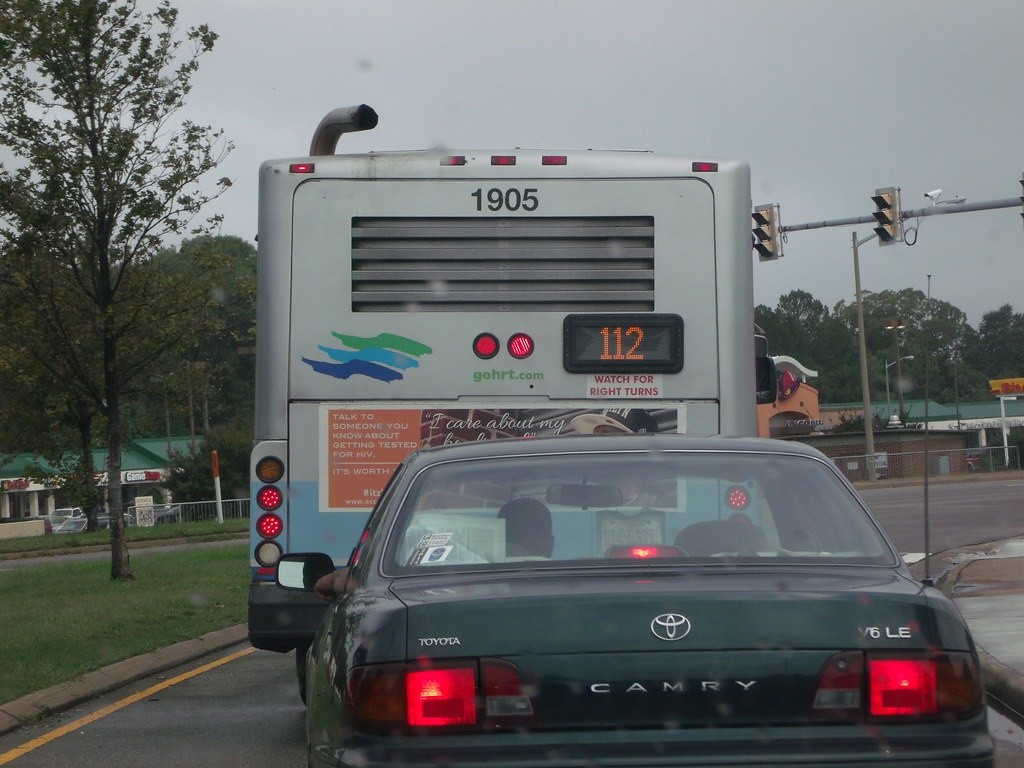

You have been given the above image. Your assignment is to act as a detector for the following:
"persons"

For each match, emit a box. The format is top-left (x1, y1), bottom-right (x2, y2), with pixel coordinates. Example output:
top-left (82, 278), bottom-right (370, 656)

top-left (570, 409), bottom-right (659, 503)
top-left (314, 499), bottom-right (554, 602)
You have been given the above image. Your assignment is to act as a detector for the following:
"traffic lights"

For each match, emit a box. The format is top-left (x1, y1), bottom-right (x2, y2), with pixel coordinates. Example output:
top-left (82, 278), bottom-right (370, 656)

top-left (871, 187), bottom-right (904, 245)
top-left (751, 203), bottom-right (783, 261)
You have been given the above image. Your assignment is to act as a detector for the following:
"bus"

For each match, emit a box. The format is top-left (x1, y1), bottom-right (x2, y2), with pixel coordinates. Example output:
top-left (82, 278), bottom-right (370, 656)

top-left (252, 103), bottom-right (775, 713)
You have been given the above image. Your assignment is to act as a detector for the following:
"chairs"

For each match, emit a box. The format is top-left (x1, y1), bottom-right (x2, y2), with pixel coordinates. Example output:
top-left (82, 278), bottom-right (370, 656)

top-left (674, 521), bottom-right (769, 556)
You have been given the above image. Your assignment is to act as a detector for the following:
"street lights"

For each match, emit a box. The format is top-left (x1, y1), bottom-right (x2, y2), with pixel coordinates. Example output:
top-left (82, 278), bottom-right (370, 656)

top-left (882, 305), bottom-right (909, 428)
top-left (884, 356), bottom-right (913, 429)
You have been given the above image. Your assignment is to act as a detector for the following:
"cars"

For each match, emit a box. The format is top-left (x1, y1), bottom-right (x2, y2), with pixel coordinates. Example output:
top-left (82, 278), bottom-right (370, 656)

top-left (22, 505), bottom-right (179, 535)
top-left (250, 435), bottom-right (992, 768)
